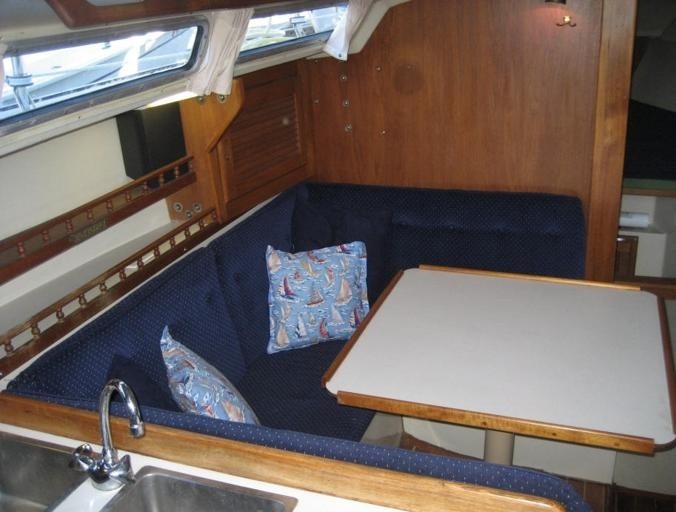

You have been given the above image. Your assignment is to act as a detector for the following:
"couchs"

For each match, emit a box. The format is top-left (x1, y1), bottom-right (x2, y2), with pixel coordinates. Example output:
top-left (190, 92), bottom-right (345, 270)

top-left (0, 178), bottom-right (590, 512)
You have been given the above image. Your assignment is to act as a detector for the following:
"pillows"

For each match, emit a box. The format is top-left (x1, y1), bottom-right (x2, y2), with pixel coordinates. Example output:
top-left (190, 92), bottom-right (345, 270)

top-left (105, 319), bottom-right (266, 428)
top-left (263, 241), bottom-right (371, 356)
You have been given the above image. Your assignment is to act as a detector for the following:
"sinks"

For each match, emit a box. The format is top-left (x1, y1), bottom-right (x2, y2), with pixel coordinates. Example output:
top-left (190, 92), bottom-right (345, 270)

top-left (0, 430), bottom-right (92, 512)
top-left (100, 465), bottom-right (298, 512)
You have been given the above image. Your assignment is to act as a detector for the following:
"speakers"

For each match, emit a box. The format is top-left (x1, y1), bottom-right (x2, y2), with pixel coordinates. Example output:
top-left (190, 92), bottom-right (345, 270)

top-left (115, 103), bottom-right (189, 188)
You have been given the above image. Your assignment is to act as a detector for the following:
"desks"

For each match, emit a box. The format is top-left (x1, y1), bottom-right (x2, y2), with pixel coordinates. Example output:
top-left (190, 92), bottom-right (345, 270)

top-left (319, 261), bottom-right (676, 468)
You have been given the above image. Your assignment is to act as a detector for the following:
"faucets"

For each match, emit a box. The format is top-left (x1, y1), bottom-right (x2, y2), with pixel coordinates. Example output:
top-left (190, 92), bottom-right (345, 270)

top-left (99, 380), bottom-right (145, 452)
